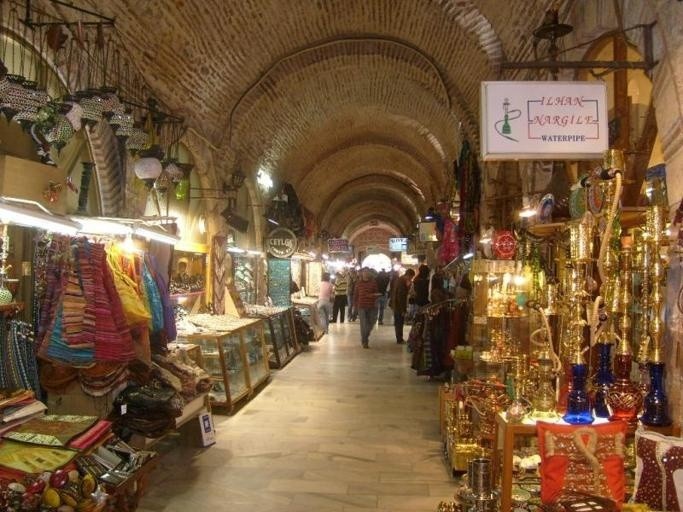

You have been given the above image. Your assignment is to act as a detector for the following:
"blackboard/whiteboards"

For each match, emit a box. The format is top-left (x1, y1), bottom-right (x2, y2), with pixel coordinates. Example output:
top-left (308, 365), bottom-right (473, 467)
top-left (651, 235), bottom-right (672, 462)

top-left (267, 258), bottom-right (291, 338)
top-left (480, 81), bottom-right (609, 159)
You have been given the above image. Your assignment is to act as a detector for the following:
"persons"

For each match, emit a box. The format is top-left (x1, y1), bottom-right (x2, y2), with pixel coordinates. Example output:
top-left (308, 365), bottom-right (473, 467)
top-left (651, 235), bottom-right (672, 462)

top-left (328, 266), bottom-right (399, 324)
top-left (430, 273), bottom-right (453, 304)
top-left (411, 263), bottom-right (429, 320)
top-left (314, 271), bottom-right (334, 335)
top-left (350, 266), bottom-right (380, 349)
top-left (388, 269), bottom-right (415, 345)
